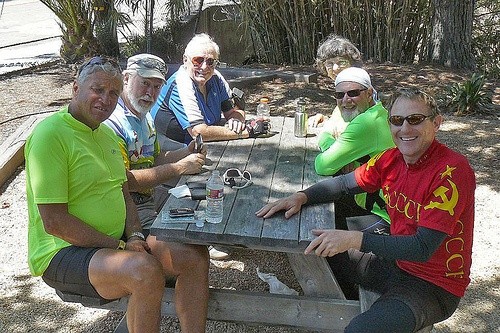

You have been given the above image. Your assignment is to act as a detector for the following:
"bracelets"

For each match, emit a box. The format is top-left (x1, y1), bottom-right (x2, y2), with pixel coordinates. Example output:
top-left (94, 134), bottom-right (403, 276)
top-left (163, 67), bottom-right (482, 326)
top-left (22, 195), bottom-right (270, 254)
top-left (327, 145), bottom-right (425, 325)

top-left (117, 240), bottom-right (126, 250)
top-left (128, 231), bottom-right (145, 241)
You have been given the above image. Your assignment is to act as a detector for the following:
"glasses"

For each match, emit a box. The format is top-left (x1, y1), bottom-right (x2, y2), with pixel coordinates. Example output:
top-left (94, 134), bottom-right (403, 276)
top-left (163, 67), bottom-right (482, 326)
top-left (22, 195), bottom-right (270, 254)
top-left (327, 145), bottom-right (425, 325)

top-left (223, 169), bottom-right (251, 189)
top-left (187, 55), bottom-right (216, 66)
top-left (323, 60), bottom-right (350, 69)
top-left (78, 57), bottom-right (122, 74)
top-left (389, 114), bottom-right (433, 126)
top-left (334, 89), bottom-right (368, 99)
top-left (129, 58), bottom-right (168, 75)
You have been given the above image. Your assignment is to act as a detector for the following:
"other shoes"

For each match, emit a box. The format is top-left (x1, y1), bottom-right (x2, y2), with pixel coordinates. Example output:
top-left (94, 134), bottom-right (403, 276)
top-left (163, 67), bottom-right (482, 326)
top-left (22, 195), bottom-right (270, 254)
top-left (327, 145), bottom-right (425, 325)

top-left (207, 245), bottom-right (233, 259)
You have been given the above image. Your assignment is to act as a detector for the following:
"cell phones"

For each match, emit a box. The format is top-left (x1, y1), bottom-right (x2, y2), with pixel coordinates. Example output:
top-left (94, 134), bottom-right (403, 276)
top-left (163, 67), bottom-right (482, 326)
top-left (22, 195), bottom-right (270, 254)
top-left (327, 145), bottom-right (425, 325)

top-left (169, 208), bottom-right (195, 218)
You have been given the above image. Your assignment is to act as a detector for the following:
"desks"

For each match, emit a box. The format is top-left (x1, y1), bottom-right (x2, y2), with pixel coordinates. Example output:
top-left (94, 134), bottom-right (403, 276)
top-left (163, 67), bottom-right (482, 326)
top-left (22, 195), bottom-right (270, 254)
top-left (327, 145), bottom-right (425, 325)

top-left (112, 115), bottom-right (360, 333)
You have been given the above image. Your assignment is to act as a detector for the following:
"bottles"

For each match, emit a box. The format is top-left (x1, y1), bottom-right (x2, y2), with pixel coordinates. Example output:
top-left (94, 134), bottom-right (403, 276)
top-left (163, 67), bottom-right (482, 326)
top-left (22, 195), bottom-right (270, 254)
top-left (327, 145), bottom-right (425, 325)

top-left (256, 98), bottom-right (270, 120)
top-left (205, 170), bottom-right (224, 224)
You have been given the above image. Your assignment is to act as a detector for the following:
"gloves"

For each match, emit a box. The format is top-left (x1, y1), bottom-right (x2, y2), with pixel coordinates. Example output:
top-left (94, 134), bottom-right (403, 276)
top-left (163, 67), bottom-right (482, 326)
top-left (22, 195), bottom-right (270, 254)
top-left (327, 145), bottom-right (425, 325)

top-left (247, 119), bottom-right (271, 138)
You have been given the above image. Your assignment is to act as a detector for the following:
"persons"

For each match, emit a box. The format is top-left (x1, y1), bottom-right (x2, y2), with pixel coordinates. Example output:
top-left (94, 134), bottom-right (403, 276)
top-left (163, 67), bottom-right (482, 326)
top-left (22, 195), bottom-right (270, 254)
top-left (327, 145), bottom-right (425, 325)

top-left (100, 52), bottom-right (206, 230)
top-left (214, 68), bottom-right (245, 110)
top-left (150, 32), bottom-right (270, 260)
top-left (306, 37), bottom-right (365, 140)
top-left (255, 86), bottom-right (477, 333)
top-left (24, 55), bottom-right (209, 333)
top-left (316, 67), bottom-right (396, 230)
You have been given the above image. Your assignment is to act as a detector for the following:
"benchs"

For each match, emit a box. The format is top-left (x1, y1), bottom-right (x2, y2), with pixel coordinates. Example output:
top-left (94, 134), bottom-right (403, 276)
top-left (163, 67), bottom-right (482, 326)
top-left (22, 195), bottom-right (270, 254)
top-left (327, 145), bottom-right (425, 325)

top-left (56, 215), bottom-right (434, 333)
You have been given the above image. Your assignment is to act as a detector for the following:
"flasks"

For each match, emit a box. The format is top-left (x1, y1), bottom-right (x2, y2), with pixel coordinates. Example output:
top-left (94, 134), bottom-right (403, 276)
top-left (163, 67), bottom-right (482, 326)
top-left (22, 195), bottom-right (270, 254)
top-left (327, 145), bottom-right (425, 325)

top-left (294, 97), bottom-right (308, 138)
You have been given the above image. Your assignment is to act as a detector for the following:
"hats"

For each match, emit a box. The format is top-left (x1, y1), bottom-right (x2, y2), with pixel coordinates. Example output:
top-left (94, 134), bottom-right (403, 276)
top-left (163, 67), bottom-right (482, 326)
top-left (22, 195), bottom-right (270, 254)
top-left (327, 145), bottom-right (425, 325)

top-left (334, 67), bottom-right (371, 89)
top-left (127, 54), bottom-right (168, 85)
